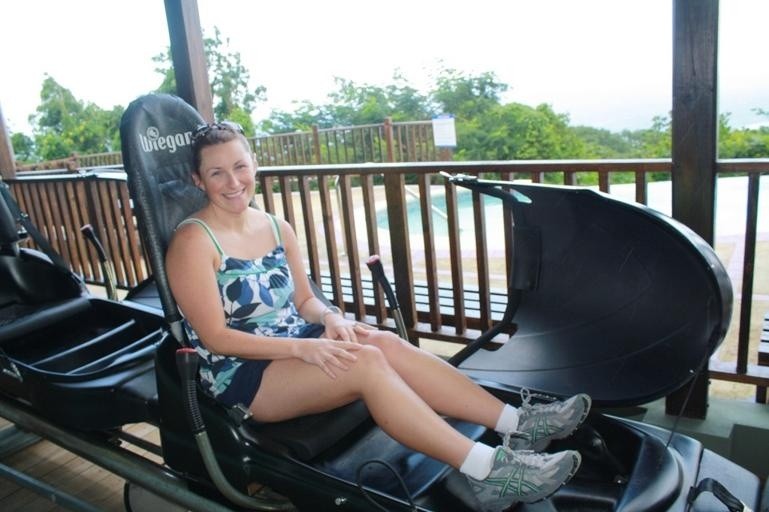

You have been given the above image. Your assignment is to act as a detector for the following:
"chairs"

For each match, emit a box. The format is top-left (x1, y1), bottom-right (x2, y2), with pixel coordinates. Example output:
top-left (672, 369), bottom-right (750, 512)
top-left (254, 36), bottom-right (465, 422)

top-left (0, 185), bottom-right (102, 414)
top-left (119, 92), bottom-right (375, 476)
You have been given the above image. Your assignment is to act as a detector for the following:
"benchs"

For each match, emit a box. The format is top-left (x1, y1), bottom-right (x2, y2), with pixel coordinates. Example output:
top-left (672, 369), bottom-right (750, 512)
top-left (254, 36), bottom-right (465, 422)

top-left (309, 272), bottom-right (517, 334)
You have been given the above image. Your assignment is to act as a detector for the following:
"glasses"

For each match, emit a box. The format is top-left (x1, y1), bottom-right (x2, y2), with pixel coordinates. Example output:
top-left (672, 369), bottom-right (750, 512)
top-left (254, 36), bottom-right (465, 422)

top-left (192, 121), bottom-right (243, 140)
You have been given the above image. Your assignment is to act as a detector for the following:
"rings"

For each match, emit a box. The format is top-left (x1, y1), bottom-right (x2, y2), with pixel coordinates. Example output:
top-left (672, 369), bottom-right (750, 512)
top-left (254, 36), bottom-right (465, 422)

top-left (353, 322), bottom-right (358, 329)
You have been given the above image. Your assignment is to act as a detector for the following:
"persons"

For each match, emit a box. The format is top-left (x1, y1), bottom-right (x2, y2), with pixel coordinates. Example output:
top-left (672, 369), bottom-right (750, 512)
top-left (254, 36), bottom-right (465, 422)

top-left (164, 121), bottom-right (593, 511)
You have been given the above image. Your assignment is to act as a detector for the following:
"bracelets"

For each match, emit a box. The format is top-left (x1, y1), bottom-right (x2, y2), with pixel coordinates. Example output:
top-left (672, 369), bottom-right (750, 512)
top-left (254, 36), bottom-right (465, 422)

top-left (319, 305), bottom-right (343, 325)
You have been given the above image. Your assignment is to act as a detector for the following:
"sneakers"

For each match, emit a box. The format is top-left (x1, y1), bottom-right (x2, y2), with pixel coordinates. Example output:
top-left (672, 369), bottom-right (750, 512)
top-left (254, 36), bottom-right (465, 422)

top-left (465, 388), bottom-right (592, 511)
